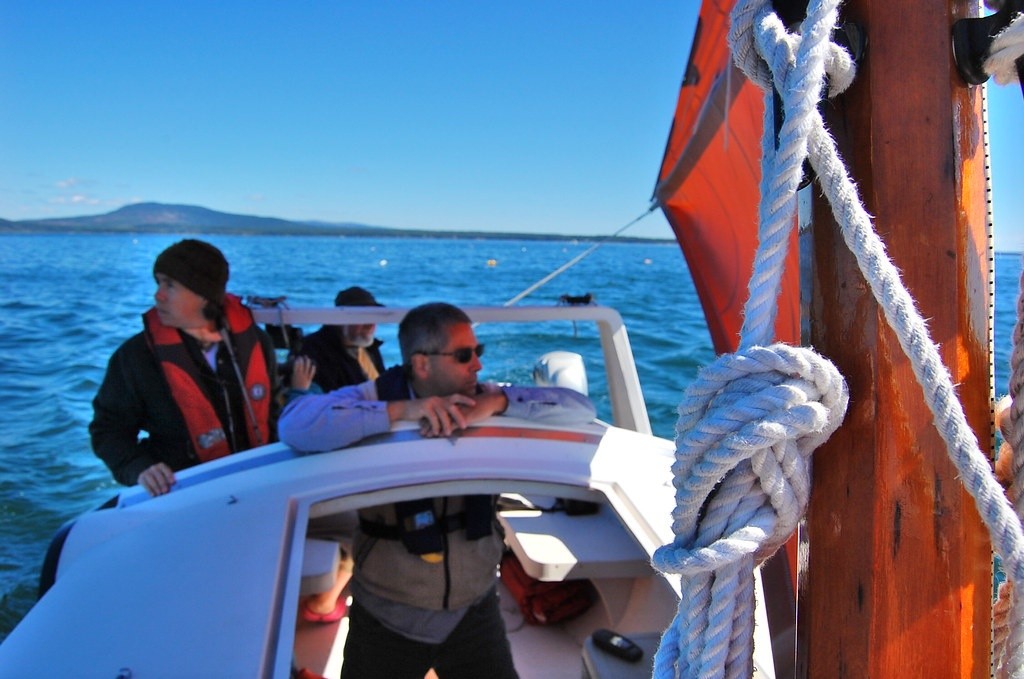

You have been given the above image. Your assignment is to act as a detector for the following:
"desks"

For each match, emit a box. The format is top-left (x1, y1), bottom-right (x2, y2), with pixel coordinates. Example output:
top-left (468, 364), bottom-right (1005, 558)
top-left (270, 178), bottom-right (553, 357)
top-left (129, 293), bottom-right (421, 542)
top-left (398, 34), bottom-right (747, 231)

top-left (500, 508), bottom-right (653, 625)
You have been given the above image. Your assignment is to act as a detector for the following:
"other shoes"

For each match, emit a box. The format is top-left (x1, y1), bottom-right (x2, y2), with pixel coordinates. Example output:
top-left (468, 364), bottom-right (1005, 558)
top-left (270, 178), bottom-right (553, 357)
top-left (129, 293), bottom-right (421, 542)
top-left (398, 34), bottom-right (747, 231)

top-left (300, 594), bottom-right (346, 622)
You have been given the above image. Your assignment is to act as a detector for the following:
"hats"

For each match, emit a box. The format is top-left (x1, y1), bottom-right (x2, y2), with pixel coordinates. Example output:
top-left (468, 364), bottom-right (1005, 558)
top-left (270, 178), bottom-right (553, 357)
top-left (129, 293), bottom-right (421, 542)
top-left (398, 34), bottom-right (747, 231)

top-left (153, 239), bottom-right (231, 304)
top-left (336, 285), bottom-right (383, 306)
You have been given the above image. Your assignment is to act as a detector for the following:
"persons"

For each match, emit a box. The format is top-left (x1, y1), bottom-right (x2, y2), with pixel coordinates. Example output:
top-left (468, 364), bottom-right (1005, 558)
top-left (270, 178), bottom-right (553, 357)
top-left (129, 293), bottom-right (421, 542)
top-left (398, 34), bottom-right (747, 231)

top-left (277, 301), bottom-right (597, 679)
top-left (88, 238), bottom-right (384, 622)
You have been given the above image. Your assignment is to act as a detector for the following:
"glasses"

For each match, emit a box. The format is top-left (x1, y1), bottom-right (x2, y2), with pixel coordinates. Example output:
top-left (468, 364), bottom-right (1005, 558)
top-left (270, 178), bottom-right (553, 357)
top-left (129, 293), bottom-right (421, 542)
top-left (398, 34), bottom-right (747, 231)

top-left (411, 343), bottom-right (485, 363)
